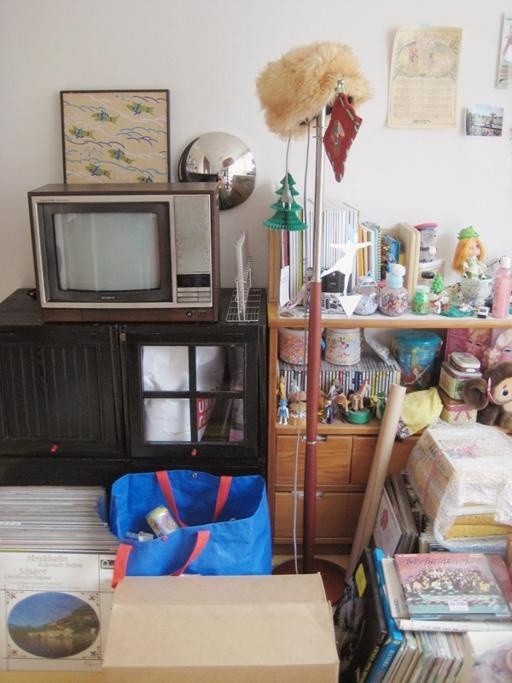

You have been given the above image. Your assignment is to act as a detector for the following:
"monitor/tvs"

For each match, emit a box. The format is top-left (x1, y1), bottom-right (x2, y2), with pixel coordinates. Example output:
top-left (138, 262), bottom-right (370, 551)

top-left (27, 184), bottom-right (220, 321)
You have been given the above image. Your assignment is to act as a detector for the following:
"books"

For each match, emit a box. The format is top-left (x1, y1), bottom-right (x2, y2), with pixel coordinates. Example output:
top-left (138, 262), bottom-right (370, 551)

top-left (333, 548), bottom-right (511, 682)
top-left (369, 421), bottom-right (511, 555)
top-left (234, 227), bottom-right (252, 314)
top-left (266, 198), bottom-right (422, 307)
top-left (1, 481), bottom-right (130, 676)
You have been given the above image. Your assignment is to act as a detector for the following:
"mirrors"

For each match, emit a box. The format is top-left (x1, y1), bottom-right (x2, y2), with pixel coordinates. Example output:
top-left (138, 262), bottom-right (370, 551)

top-left (180, 131), bottom-right (257, 211)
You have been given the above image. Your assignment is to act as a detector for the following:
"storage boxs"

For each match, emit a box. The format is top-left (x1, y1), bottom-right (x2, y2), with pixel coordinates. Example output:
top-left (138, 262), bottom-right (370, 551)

top-left (101, 571), bottom-right (339, 683)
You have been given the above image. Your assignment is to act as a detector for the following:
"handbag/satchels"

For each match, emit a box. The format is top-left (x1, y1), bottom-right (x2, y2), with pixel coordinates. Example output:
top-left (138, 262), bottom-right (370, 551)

top-left (109, 470), bottom-right (272, 575)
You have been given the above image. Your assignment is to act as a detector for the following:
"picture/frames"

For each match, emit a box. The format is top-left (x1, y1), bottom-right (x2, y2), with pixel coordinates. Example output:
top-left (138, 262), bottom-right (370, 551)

top-left (60, 88), bottom-right (172, 185)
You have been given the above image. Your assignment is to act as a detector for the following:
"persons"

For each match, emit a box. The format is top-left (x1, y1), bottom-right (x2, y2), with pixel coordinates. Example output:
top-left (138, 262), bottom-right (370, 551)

top-left (483, 328), bottom-right (511, 379)
top-left (449, 224), bottom-right (486, 283)
top-left (462, 327), bottom-right (491, 364)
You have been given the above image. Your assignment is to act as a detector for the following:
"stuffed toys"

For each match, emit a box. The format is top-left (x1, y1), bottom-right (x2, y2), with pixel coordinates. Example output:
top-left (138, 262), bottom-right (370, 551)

top-left (462, 361), bottom-right (511, 437)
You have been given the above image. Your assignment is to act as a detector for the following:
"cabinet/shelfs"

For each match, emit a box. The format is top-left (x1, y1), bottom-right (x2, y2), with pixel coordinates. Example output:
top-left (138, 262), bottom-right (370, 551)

top-left (0, 286), bottom-right (267, 486)
top-left (265, 288), bottom-right (512, 547)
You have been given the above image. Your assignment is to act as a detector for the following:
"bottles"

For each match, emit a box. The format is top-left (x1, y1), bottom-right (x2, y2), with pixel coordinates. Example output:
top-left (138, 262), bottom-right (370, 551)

top-left (491, 255), bottom-right (512, 319)
top-left (411, 270), bottom-right (436, 315)
top-left (350, 275), bottom-right (378, 316)
top-left (379, 273), bottom-right (409, 317)
